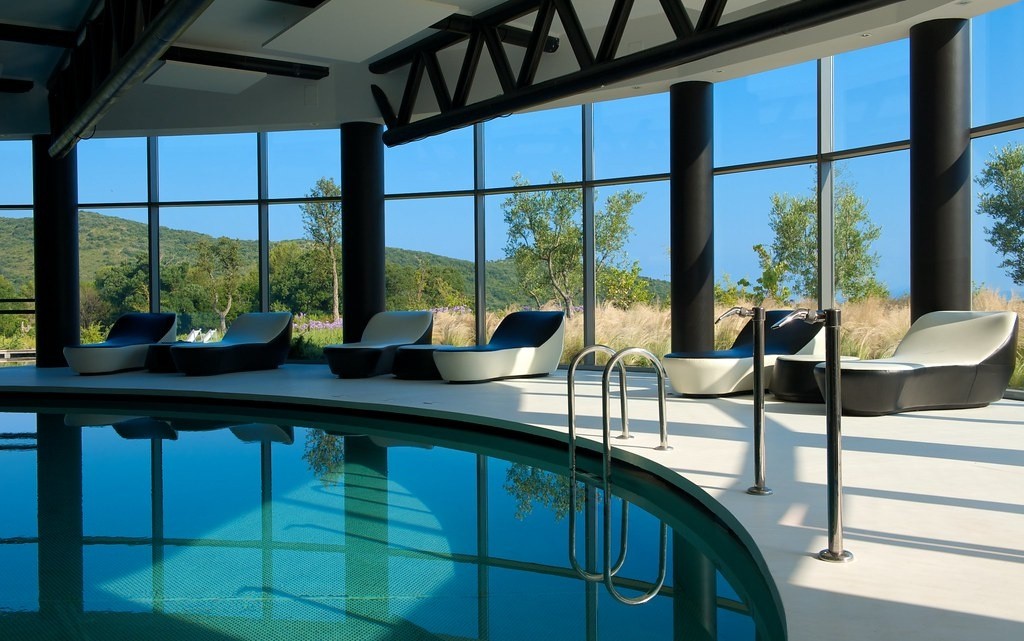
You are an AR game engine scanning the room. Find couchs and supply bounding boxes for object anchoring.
[170,311,292,373]
[813,311,1019,416]
[63,310,178,373]
[434,310,565,381]
[324,311,434,376]
[663,310,826,397]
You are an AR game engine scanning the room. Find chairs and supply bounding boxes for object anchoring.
[184,329,217,343]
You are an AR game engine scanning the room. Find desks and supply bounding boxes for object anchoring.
[391,345,454,381]
[770,356,858,403]
[145,340,189,373]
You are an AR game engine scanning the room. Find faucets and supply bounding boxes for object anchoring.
[771,309,859,565]
[715,306,772,496]
